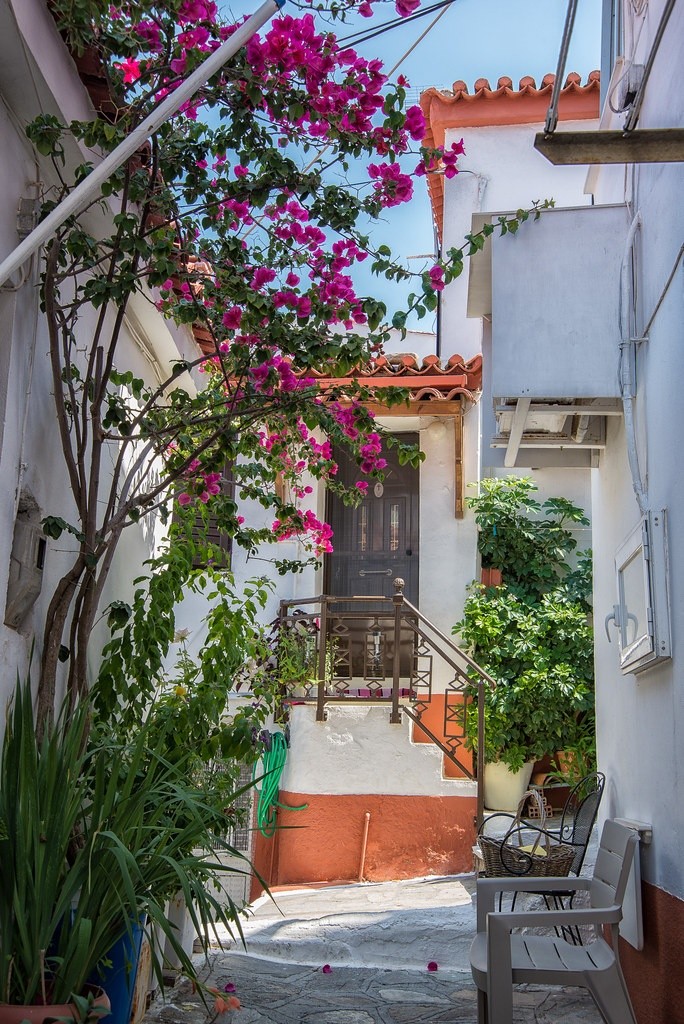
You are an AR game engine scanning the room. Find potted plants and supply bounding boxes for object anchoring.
[547,707,595,784]
[465,474,590,587]
[451,581,594,812]
[1,624,340,1023]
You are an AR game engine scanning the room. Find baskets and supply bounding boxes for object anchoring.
[478,789,576,877]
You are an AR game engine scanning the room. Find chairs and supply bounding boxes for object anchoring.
[469,819,640,1022]
[477,771,606,946]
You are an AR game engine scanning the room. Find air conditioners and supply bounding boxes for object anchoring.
[494,397,574,439]
